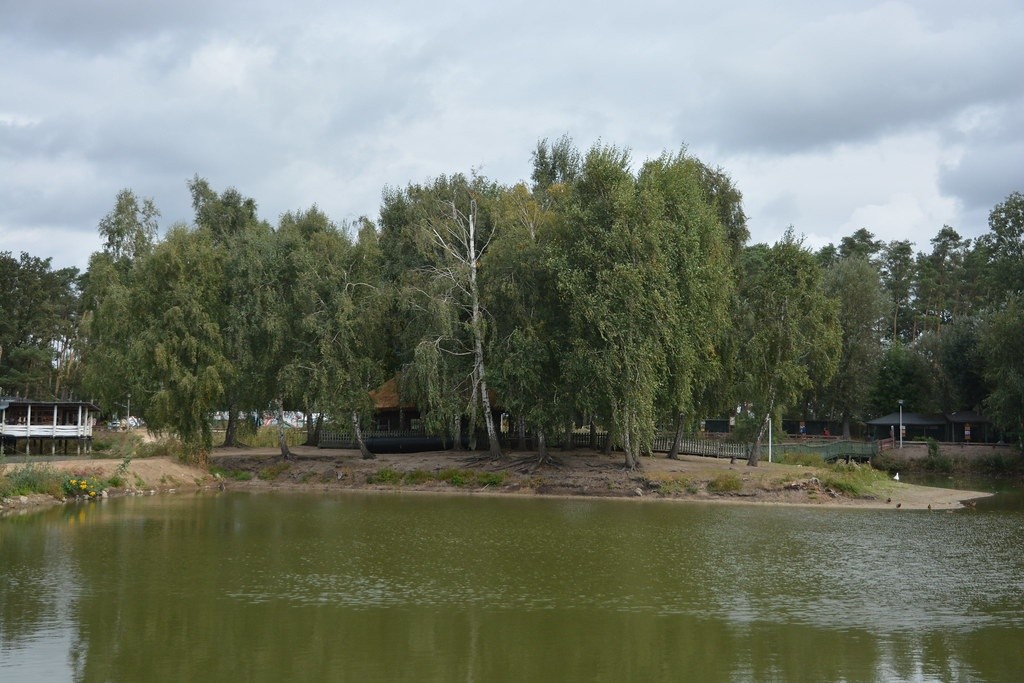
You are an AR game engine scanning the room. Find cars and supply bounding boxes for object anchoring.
[207,410,330,427]
[106,416,145,431]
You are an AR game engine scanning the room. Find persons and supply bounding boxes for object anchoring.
[802,425,807,435]
[823,428,830,439]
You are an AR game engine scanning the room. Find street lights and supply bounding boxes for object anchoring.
[896,398,905,448]
[113,394,131,431]
